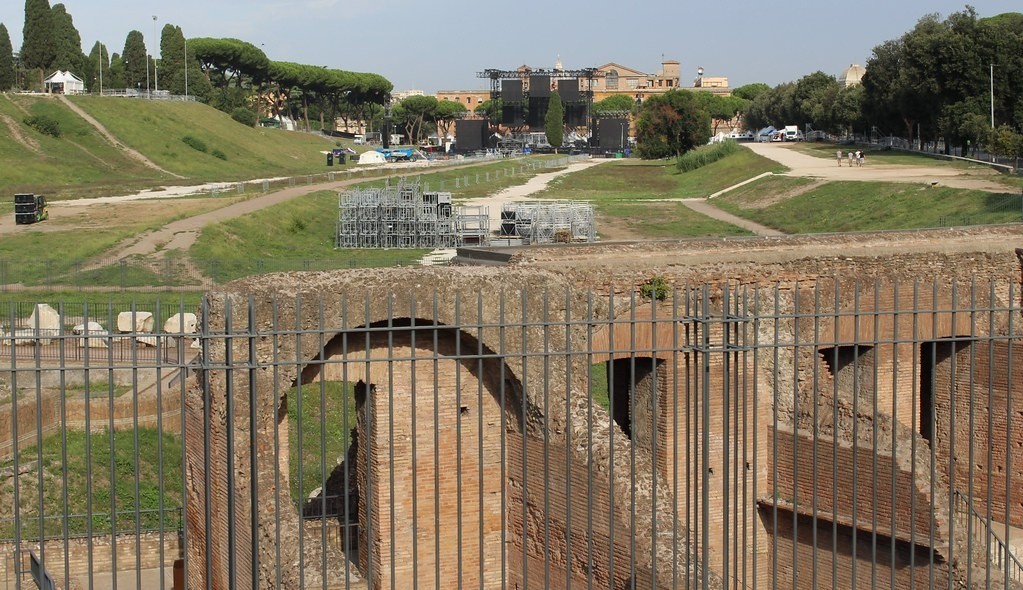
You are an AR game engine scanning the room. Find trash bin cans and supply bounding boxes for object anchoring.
[339,152,347,164]
[326,153,334,166]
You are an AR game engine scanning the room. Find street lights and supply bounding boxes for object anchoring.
[990,64,1000,129]
[99,41,103,95]
[620,123,625,148]
[153,15,158,92]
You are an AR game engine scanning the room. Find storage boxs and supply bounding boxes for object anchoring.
[15,193,35,224]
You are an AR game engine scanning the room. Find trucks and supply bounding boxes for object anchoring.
[354,135,366,145]
[785,125,798,142]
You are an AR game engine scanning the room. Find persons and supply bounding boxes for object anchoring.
[848,150,865,167]
[837,150,842,167]
